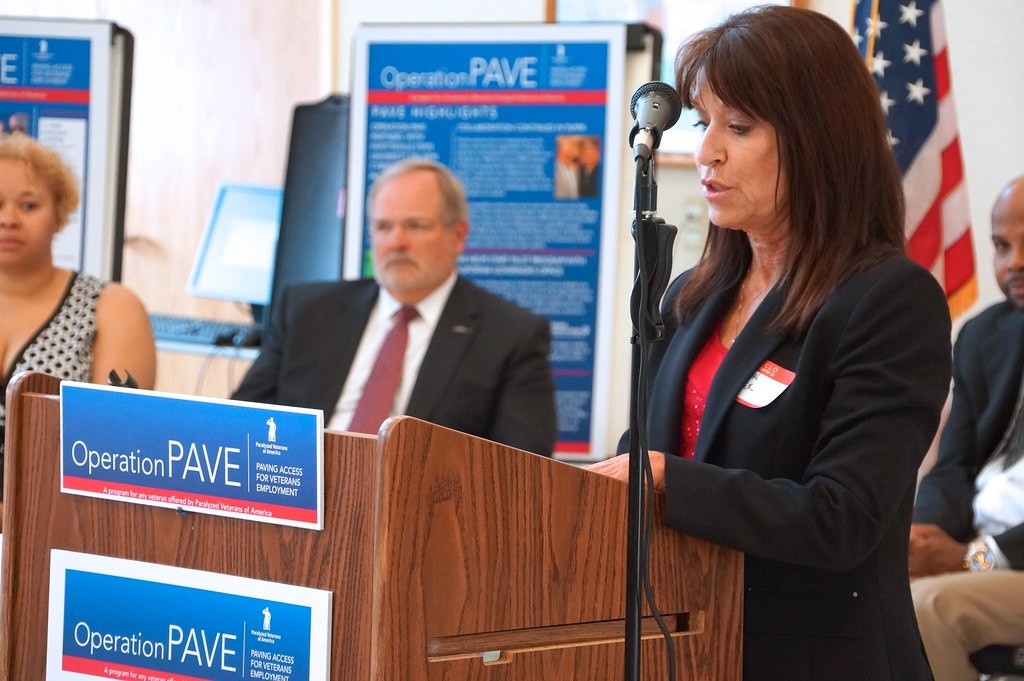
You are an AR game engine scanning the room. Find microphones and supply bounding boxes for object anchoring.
[630,81,682,164]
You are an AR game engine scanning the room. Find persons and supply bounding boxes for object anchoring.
[0,131,157,535]
[584,5,952,681]
[227,158,557,458]
[908,175,1024,681]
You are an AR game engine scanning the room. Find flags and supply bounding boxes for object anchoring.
[852,0,978,322]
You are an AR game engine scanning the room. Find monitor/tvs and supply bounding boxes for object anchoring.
[187,183,283,320]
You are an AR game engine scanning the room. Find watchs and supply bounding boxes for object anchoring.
[962,540,998,572]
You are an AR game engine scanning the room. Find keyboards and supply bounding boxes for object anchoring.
[147,311,241,345]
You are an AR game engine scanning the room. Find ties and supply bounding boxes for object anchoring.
[346,305,423,435]
[986,378,1024,472]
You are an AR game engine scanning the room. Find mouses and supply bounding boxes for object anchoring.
[234,325,263,347]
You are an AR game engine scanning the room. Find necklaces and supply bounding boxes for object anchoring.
[732,263,771,343]
